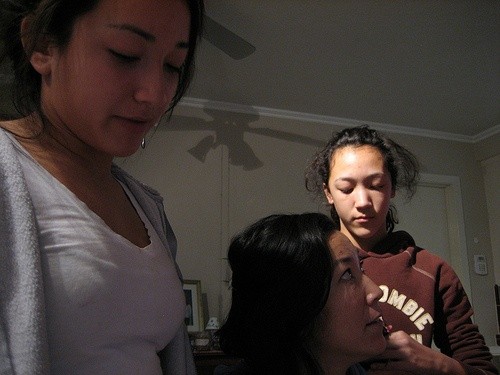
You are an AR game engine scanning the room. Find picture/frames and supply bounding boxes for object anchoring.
[182,280,204,334]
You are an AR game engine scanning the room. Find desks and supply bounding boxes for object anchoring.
[189,348,226,375]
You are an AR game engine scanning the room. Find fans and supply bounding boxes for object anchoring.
[201,14,257,62]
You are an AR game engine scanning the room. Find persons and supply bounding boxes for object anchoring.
[211,212,391,375]
[305,120,499,375]
[1,0,206,375]
